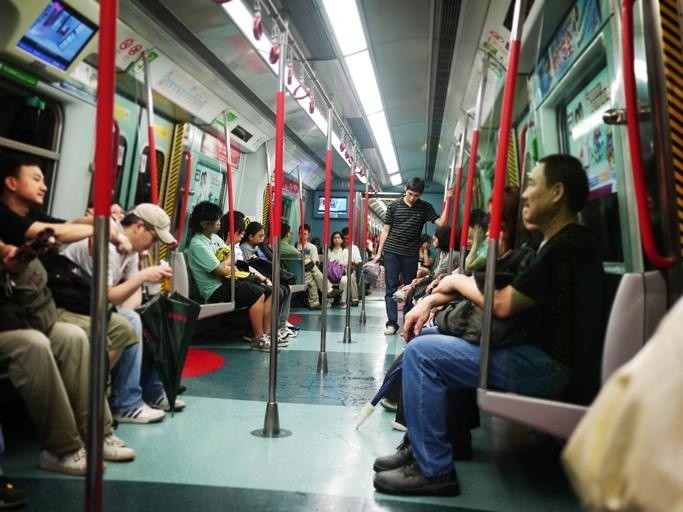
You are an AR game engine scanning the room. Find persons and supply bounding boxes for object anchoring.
[187,200,300,351]
[278,221,361,308]
[365,174,487,338]
[367,151,611,496]
[0,157,138,478]
[381,183,540,430]
[0,235,30,511]
[47,201,188,422]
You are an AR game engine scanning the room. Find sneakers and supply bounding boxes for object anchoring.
[310,289,359,311]
[38,384,186,476]
[244,320,300,352]
[384,325,395,335]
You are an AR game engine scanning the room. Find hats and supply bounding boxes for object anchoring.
[130,201,177,245]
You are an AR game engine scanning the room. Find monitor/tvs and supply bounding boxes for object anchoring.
[5,0,100,83]
[313,191,349,218]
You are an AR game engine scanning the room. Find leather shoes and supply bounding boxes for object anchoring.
[373,396,473,496]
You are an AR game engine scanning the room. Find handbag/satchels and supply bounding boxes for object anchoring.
[434,286,528,350]
[413,277,432,299]
[215,247,261,286]
[249,258,297,285]
[0,256,56,333]
[328,261,345,283]
[42,255,92,317]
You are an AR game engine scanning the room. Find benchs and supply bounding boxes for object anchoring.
[172,246,346,319]
[477,269,667,440]
